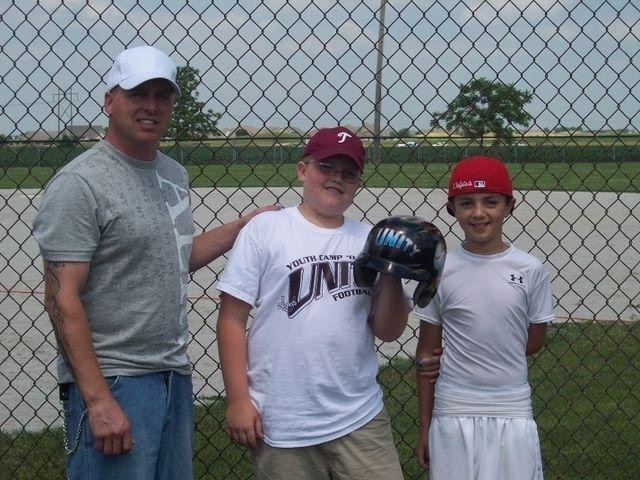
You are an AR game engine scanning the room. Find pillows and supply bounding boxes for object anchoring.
[350,209,447,309]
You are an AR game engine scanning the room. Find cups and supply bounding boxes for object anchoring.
[304,157,364,184]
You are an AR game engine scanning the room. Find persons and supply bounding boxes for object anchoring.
[414,154,554,480]
[37,43,288,478]
[215,125,415,478]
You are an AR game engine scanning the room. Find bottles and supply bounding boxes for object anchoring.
[444,153,516,219]
[301,125,367,175]
[102,44,185,102]
